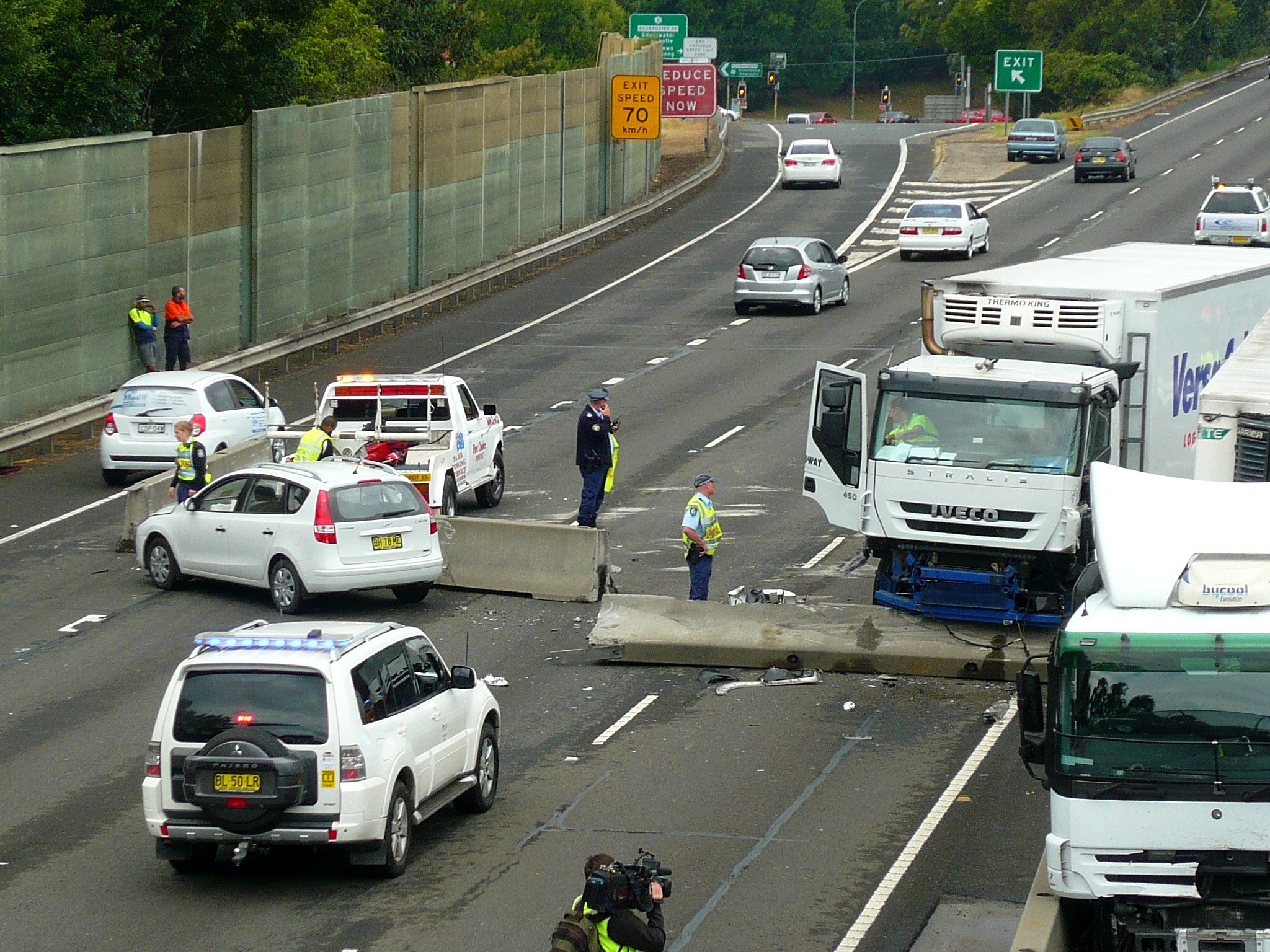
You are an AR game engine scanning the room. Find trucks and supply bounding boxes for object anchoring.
[800,241,1270,629]
[1013,302,1270,952]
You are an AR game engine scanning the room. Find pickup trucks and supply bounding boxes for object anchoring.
[309,373,505,517]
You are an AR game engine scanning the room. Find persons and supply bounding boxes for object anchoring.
[293,415,337,463]
[255,484,282,512]
[129,294,162,373]
[163,286,194,371]
[571,854,666,952]
[884,397,945,446]
[681,473,723,600]
[168,421,205,581]
[575,387,619,531]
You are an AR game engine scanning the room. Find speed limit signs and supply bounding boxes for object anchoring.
[609,74,661,141]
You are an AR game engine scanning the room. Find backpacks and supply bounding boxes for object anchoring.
[551,900,613,952]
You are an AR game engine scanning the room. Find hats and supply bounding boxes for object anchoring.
[588,387,609,401]
[694,474,716,488]
[135,294,150,302]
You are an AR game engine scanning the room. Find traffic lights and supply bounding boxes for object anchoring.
[767,71,775,85]
[737,84,746,99]
[881,90,890,104]
[955,72,962,86]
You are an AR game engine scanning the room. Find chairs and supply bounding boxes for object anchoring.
[249,484,281,513]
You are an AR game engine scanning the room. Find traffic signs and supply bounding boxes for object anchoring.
[718,62,763,77]
[994,49,1044,91]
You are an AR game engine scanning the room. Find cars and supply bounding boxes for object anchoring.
[875,110,922,124]
[787,112,837,124]
[1006,118,1066,162]
[1073,136,1138,183]
[898,198,991,261]
[944,107,1013,123]
[779,138,847,189]
[100,369,286,486]
[719,107,740,123]
[733,236,850,314]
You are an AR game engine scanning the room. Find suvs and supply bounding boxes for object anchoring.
[140,617,501,878]
[134,455,446,615]
[1194,174,1270,248]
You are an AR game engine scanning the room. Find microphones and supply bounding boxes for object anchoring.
[648,868,672,875]
